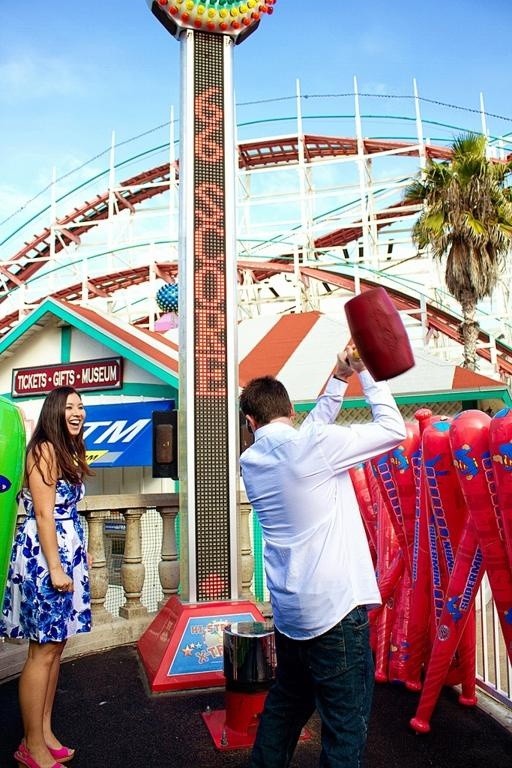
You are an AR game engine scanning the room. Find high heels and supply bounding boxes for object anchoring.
[12,736,75,767]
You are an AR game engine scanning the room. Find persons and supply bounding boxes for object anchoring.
[236,342,409,767]
[0,384,100,768]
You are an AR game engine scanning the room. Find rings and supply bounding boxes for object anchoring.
[57,587,63,593]
